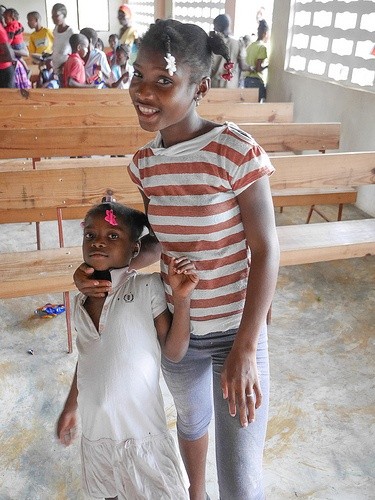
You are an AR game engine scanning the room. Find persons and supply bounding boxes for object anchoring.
[0,4,269,102]
[55,201,200,500]
[71,19,280,500]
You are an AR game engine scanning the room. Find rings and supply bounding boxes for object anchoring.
[247,394,254,397]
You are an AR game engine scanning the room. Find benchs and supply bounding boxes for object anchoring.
[0,88,375,354]
